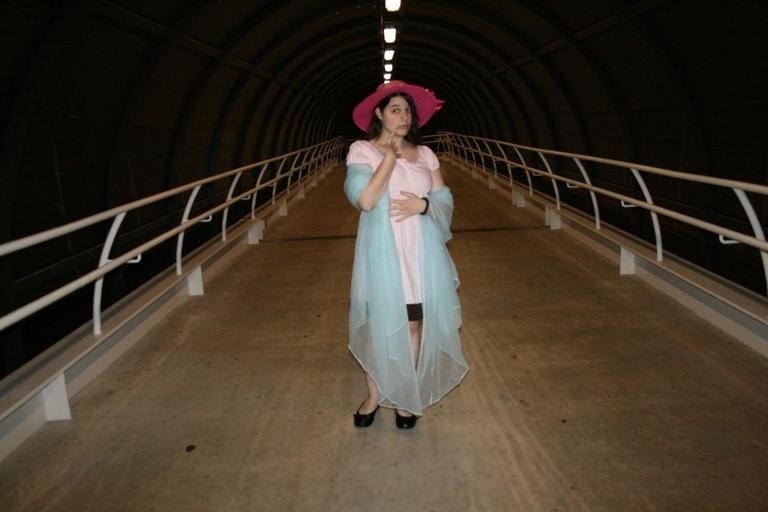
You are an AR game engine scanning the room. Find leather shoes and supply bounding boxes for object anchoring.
[353,399,380,427]
[395,409,416,429]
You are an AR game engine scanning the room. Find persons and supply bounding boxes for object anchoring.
[344,80,470,429]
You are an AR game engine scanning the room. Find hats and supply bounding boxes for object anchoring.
[351,80,445,134]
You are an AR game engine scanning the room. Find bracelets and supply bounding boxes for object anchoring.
[421,198,429,215]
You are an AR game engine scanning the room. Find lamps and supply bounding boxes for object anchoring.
[383,0,402,15]
[382,63,394,85]
[382,48,395,61]
[382,26,397,44]
